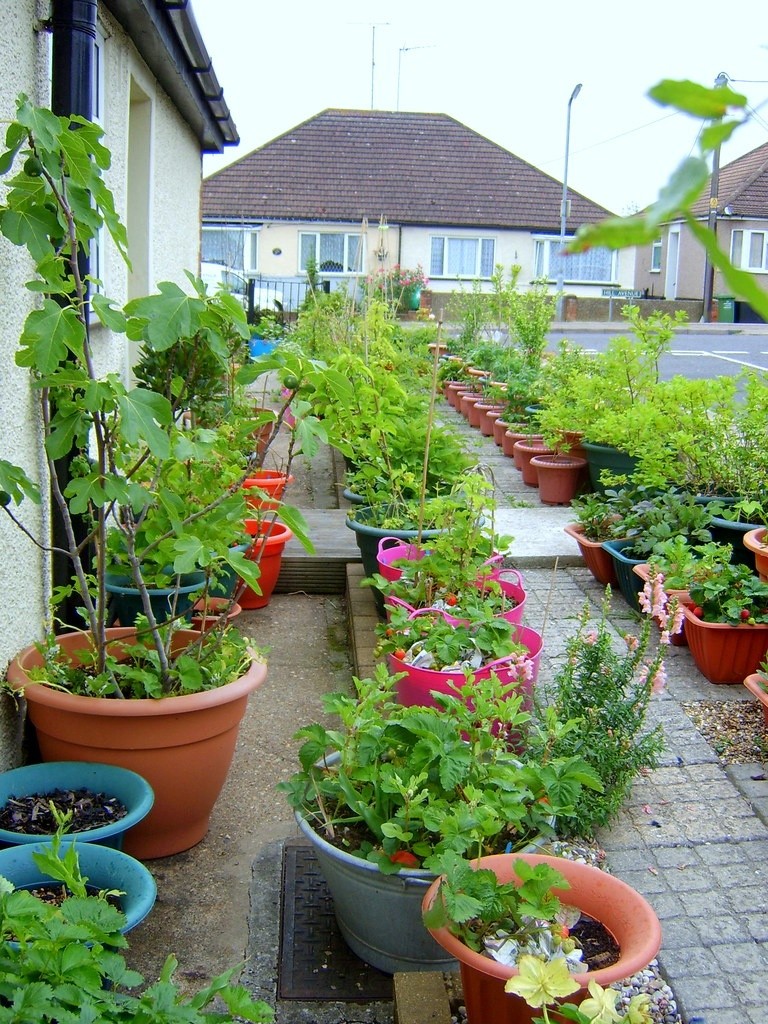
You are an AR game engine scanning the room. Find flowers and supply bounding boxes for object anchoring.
[376,263,428,292]
[504,955,654,1024]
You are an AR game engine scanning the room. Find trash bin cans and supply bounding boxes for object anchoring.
[713,295,736,323]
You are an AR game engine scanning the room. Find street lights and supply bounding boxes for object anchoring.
[553,84,585,317]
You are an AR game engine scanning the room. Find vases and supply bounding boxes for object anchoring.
[404,288,421,310]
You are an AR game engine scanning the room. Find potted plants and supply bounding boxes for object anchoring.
[0,88,768,1024]
